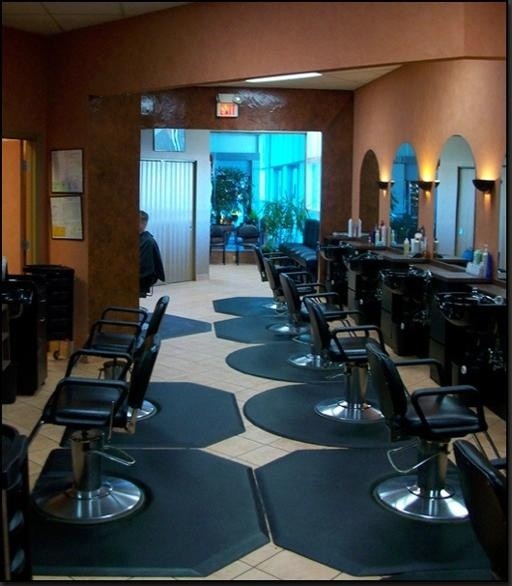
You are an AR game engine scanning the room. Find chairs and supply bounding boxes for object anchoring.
[367,341,489,524]
[235,222,263,266]
[304,296,388,424]
[254,246,312,310]
[72,295,169,424]
[263,258,331,335]
[280,273,360,369]
[210,224,228,265]
[26,337,163,525]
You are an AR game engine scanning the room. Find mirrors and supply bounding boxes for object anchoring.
[357,149,378,234]
[433,135,476,263]
[389,141,420,249]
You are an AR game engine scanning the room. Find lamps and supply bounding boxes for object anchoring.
[378,178,395,192]
[415,178,439,191]
[474,174,498,195]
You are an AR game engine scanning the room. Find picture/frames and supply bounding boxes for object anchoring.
[51,148,83,194]
[51,194,85,241]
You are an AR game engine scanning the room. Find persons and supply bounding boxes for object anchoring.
[138,210,166,298]
